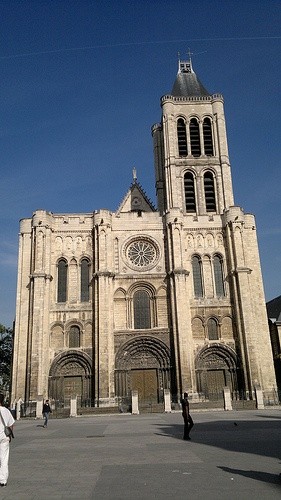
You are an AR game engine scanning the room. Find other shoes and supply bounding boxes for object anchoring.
[0,483,8,488]
[43,425,48,428]
[183,437,191,440]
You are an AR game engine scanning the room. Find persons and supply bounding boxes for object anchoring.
[43,400,53,428]
[182,392,194,440]
[0,394,16,487]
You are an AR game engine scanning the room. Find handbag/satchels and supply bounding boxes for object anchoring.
[5,427,14,440]
